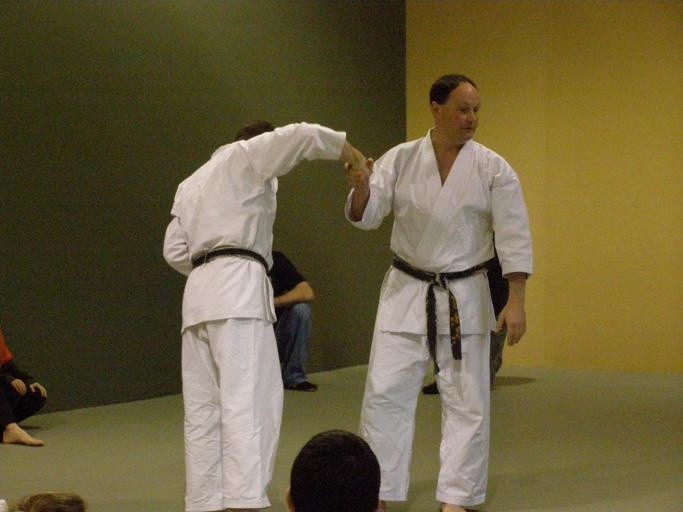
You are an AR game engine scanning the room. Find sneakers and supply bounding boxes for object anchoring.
[285,379,317,391]
[423,381,440,394]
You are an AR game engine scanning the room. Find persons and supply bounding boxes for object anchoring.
[342,73,533,512]
[163,119,372,512]
[0,329,47,447]
[9,491,89,512]
[284,428,382,512]
[266,250,319,392]
[422,256,510,395]
